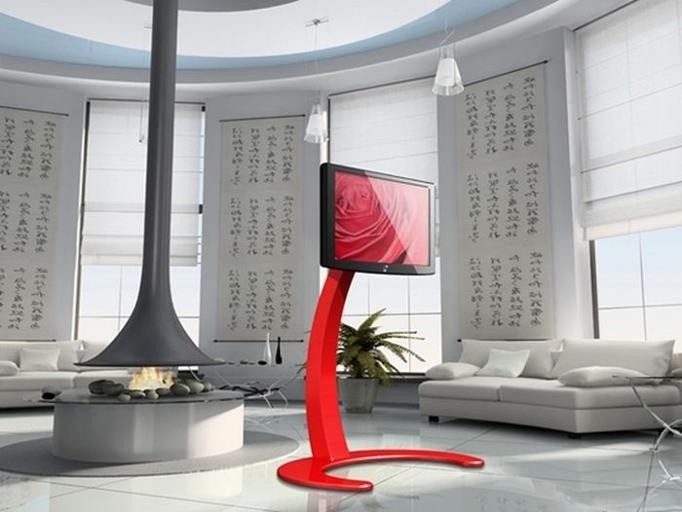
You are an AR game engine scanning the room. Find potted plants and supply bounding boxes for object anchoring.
[285,307,426,414]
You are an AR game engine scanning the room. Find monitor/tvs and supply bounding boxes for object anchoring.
[320,162,437,275]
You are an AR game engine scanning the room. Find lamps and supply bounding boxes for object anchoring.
[431,13,465,97]
[304,20,329,144]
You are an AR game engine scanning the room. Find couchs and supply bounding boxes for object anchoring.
[0,340,179,408]
[418,338,682,440]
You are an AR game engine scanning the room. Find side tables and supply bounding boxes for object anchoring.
[608,374,682,511]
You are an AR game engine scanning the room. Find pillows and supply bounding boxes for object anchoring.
[425,339,676,387]
[0,339,178,376]
